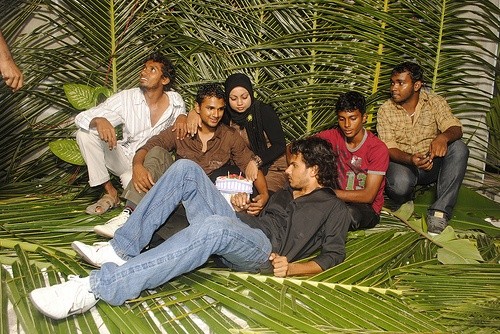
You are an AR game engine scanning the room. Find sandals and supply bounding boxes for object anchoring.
[86,190,120,215]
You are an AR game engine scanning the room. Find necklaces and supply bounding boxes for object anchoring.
[410,112,415,117]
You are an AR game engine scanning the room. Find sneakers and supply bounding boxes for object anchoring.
[94,208,132,238]
[425,209,448,236]
[71,240,127,267]
[29,274,100,320]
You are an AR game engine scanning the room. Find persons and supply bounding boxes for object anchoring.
[376,61,469,234]
[0,34,23,91]
[30,137,350,320]
[93,73,285,253]
[286,91,389,231]
[75,55,190,217]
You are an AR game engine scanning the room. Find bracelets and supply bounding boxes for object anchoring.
[250,155,262,166]
